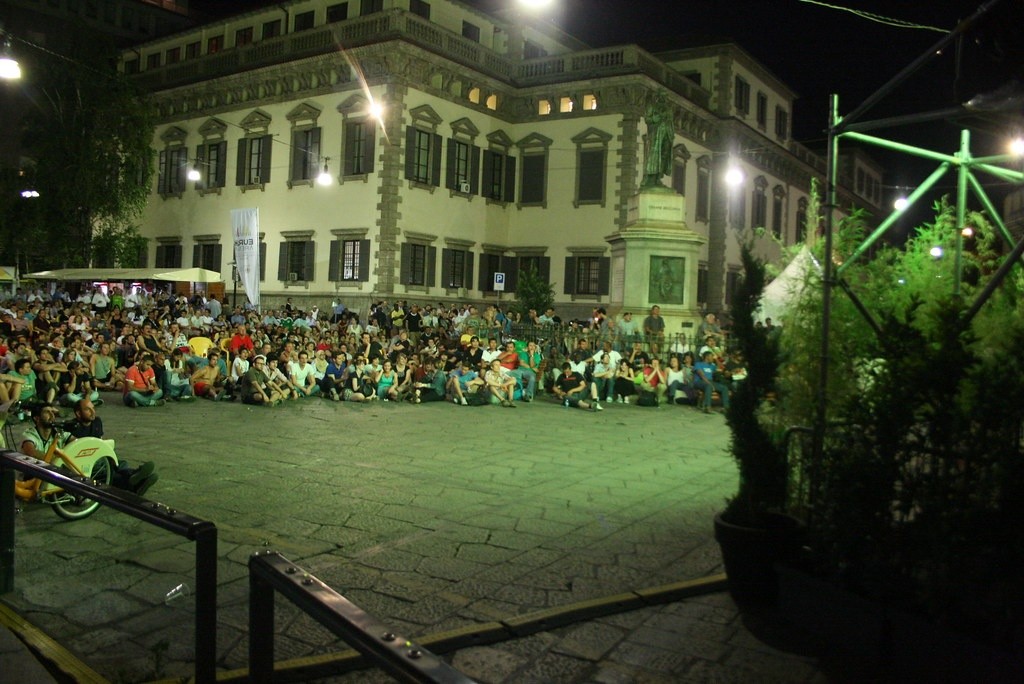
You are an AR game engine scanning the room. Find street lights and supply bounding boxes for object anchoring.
[228,260,239,309]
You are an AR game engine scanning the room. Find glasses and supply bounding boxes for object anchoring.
[256,362,265,366]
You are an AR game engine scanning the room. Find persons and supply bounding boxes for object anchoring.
[59,399,159,497]
[0,283,773,425]
[12,403,77,482]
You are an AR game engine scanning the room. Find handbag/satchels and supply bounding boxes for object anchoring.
[361,379,373,397]
[638,390,658,407]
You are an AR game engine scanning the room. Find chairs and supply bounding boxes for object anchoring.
[221,337,233,367]
[187,337,214,359]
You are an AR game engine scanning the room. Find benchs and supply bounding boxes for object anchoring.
[696,389,775,411]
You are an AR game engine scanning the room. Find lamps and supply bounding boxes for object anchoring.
[0,38,20,70]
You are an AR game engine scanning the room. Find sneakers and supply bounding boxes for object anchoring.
[92,399,104,406]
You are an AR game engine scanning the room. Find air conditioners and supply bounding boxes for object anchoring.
[290,273,298,281]
[253,176,260,184]
[460,183,470,193]
[457,288,468,298]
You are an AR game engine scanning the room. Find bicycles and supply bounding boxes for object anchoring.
[1,403,120,521]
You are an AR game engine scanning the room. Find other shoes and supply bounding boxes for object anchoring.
[523,395,532,402]
[214,389,226,401]
[154,400,166,406]
[129,461,159,496]
[501,400,516,407]
[223,395,237,402]
[362,396,377,404]
[333,394,339,401]
[577,400,589,409]
[704,405,712,413]
[412,393,417,402]
[591,402,603,410]
[454,394,458,403]
[263,400,273,408]
[690,399,697,406]
[667,395,675,404]
[180,395,196,403]
[597,397,600,401]
[617,396,629,404]
[274,399,282,405]
[460,397,468,406]
[606,396,613,403]
